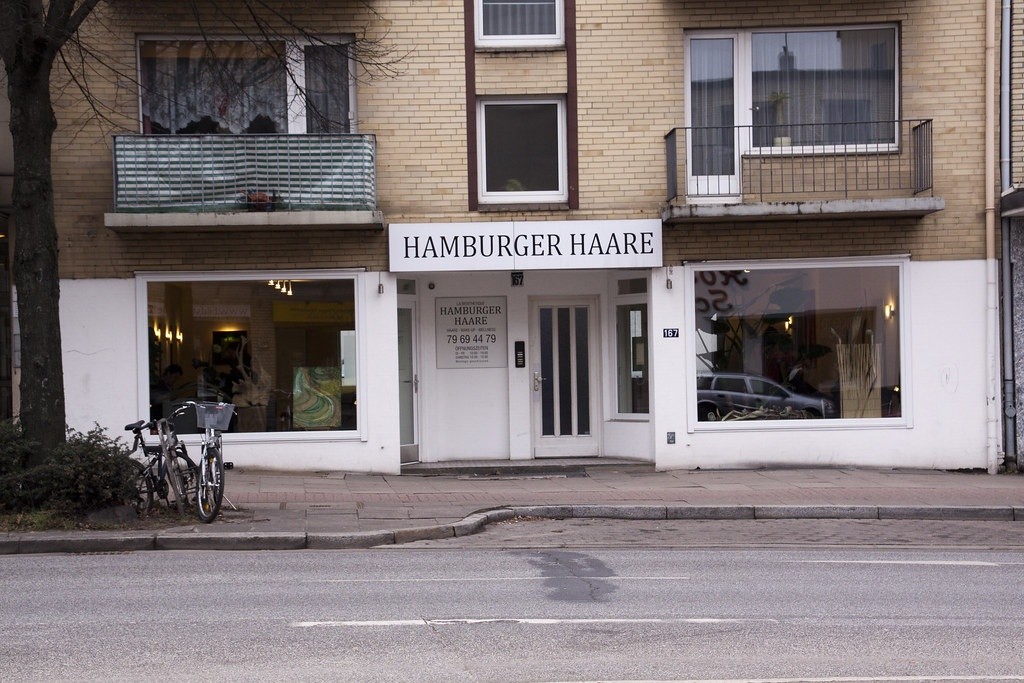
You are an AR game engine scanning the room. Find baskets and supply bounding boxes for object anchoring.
[195,404,234,430]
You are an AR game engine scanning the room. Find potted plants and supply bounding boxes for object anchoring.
[767,89,794,149]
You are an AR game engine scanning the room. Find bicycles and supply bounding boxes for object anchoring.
[124,405,200,516]
[173,400,238,523]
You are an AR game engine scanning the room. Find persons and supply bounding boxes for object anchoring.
[157,365,199,433]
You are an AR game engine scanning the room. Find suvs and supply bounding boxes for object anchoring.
[697,372,835,421]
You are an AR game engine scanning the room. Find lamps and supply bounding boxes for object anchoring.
[268,280,293,296]
[885,305,895,321]
